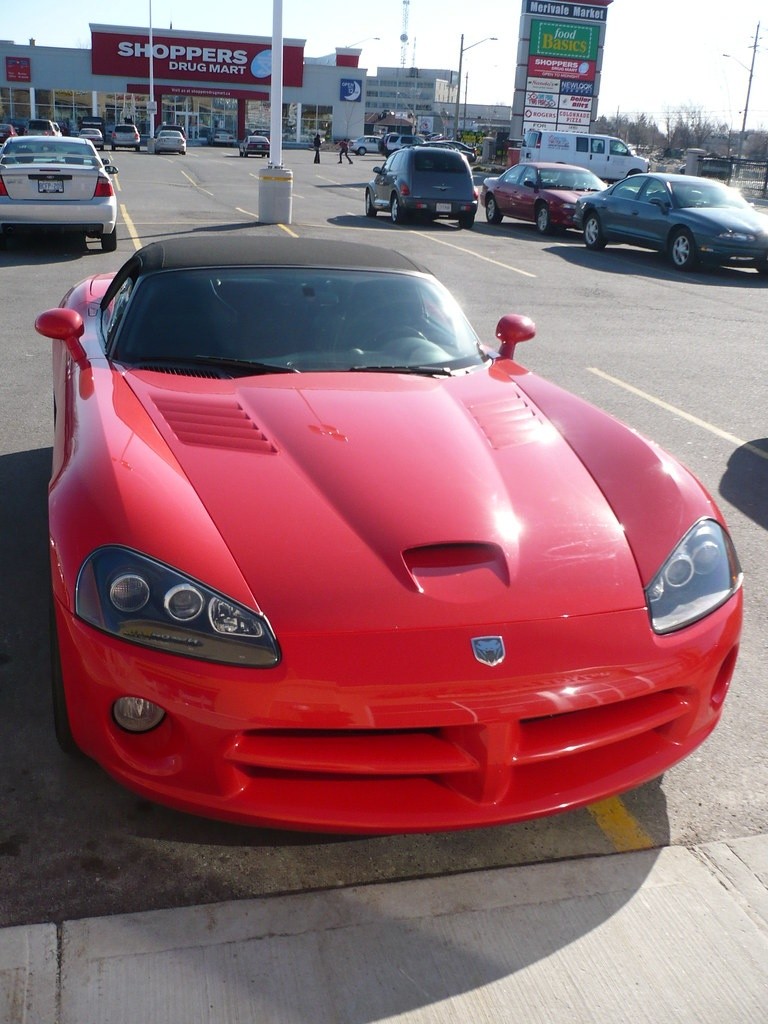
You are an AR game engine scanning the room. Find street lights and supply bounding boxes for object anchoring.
[722,53,753,180]
[452,34,499,142]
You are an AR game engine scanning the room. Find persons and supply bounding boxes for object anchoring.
[335,141,353,164]
[314,134,321,163]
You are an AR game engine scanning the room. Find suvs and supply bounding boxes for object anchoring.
[108,123,142,152]
[160,125,187,141]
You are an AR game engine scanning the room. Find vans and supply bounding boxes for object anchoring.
[519,129,652,184]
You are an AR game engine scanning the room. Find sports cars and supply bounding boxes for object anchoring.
[34,231,745,836]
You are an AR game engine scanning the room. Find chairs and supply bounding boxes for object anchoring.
[211,278,279,360]
[66,151,84,164]
[597,143,602,152]
[15,148,34,163]
[334,280,412,353]
[689,191,702,202]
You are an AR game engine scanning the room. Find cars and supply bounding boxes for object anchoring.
[479,161,611,236]
[0,123,19,144]
[679,158,732,180]
[569,171,768,279]
[364,146,479,229]
[238,135,270,159]
[250,129,270,143]
[23,115,106,137]
[349,135,382,156]
[153,130,187,155]
[0,135,118,252]
[189,123,328,142]
[75,128,105,151]
[6,118,28,135]
[208,130,236,148]
[382,130,480,164]
